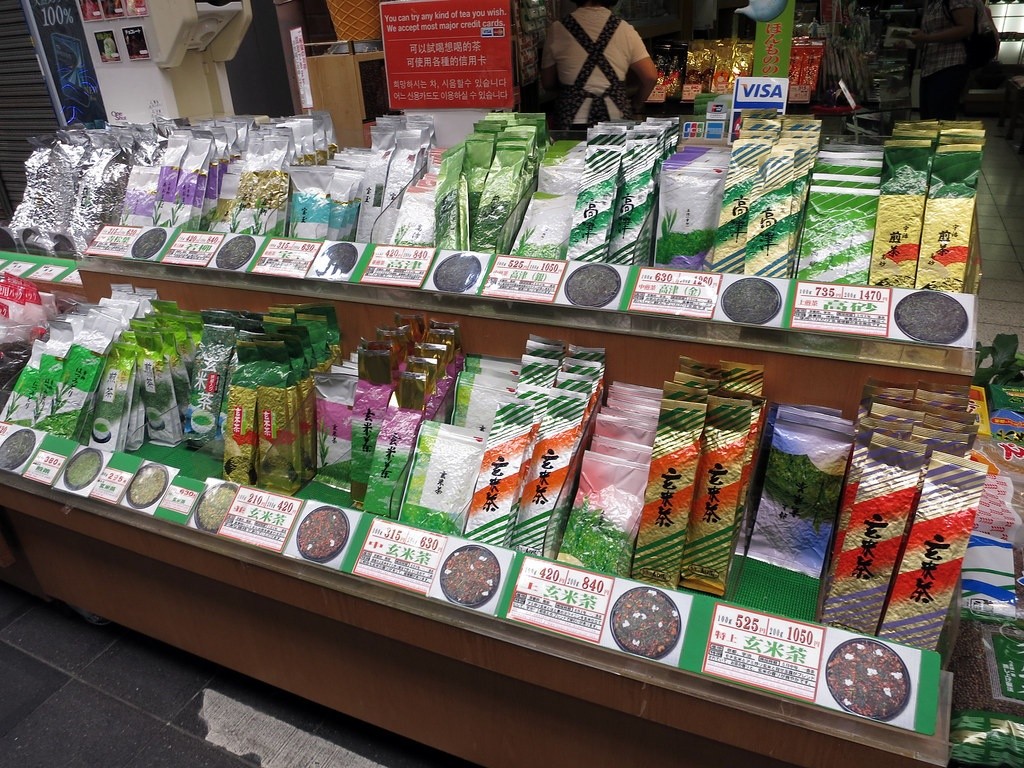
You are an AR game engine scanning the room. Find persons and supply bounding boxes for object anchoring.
[906,0,983,127]
[540,0,659,138]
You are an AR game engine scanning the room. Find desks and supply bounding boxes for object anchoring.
[302,49,393,148]
